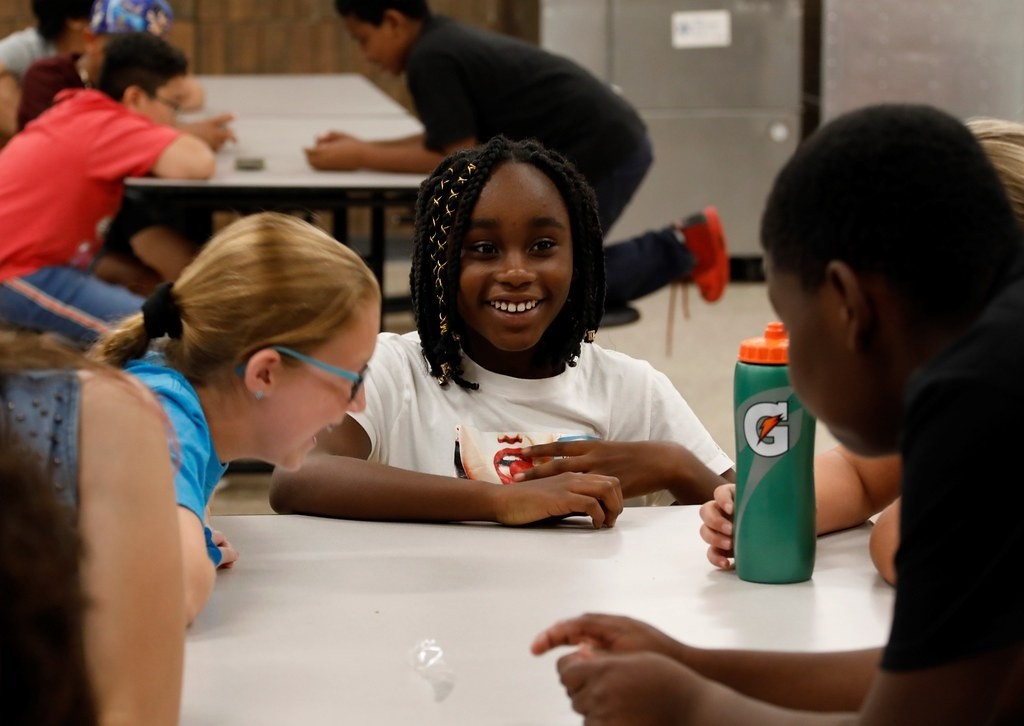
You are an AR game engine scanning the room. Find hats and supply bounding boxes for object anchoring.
[90,0,174,38]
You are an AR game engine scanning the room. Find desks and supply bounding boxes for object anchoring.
[123,73,428,334]
[181,503,898,726]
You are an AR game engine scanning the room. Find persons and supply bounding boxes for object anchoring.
[699,116,1024,589]
[530,99,1024,726]
[0,321,189,726]
[17,0,174,130]
[0,28,240,352]
[0,0,87,73]
[302,0,731,309]
[27,208,384,632]
[267,133,737,528]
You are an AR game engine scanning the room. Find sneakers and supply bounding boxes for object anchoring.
[680,206,729,302]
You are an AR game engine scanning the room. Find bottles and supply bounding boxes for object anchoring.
[734,321,818,583]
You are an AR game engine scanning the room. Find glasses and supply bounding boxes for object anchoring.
[145,92,183,114]
[234,345,369,402]
